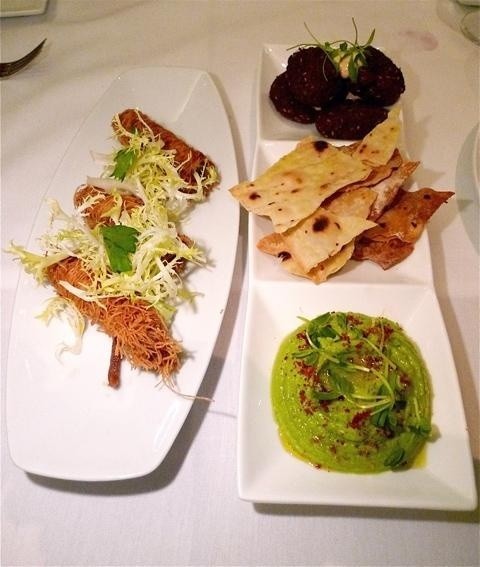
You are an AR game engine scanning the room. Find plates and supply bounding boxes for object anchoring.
[4,67,241,483]
[246,43,478,515]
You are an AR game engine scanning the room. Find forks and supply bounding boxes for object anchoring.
[1,39,45,79]
